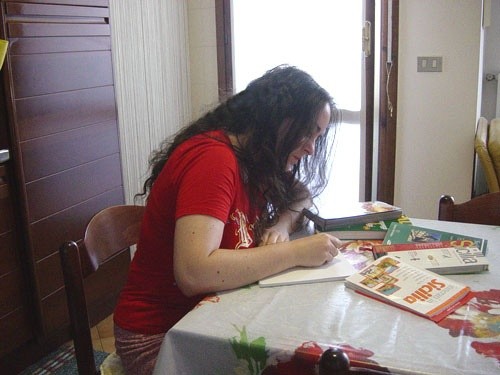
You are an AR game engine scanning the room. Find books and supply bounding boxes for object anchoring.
[259,201,490,324]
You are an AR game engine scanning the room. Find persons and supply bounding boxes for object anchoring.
[113,66,343,375]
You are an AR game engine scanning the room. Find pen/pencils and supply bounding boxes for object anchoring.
[316,229,343,257]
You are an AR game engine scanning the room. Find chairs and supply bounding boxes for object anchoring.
[58,205,145,375]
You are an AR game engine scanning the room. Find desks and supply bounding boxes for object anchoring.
[171,216,500,375]
